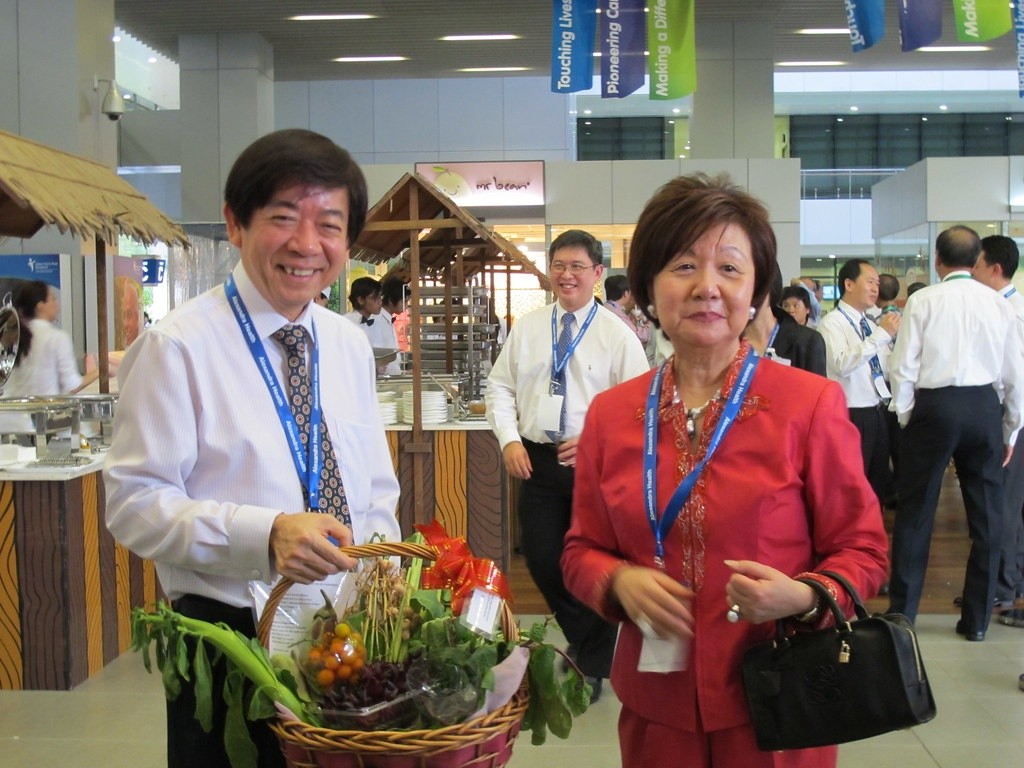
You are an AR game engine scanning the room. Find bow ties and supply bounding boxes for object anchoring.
[392,317,396,322]
[360,315,374,327]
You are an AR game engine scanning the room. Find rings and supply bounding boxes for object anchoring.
[727,604,739,623]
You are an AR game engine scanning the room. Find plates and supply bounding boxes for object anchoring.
[376,391,454,426]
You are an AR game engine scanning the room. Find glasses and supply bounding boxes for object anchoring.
[549,263,597,275]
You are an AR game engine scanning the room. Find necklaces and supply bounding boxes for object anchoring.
[671,383,723,438]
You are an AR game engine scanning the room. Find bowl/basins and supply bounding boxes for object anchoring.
[469,401,485,414]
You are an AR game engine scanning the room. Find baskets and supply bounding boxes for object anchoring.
[256,540,530,768]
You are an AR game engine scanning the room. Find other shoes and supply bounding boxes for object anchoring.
[1001,609,1024,627]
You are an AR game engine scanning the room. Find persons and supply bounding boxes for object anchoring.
[621,293,644,328]
[0,279,117,400]
[475,296,508,343]
[602,274,651,350]
[342,277,397,379]
[367,279,411,375]
[777,285,817,331]
[314,286,331,308]
[907,281,927,300]
[143,311,152,329]
[873,273,903,343]
[953,236,1024,608]
[484,230,651,706]
[425,298,459,340]
[871,225,1024,641]
[791,277,823,324]
[816,259,901,597]
[560,167,889,768]
[102,128,402,768]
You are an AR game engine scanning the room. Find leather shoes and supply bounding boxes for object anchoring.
[954,596,1013,608]
[955,619,984,641]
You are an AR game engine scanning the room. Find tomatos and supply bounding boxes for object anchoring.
[305,623,370,688]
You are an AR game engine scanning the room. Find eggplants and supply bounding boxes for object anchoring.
[314,590,338,638]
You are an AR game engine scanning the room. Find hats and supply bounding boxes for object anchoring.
[322,286,332,299]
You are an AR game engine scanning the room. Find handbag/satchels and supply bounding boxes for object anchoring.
[741,569,937,752]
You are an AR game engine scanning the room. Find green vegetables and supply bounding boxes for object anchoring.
[128,600,320,768]
[409,616,593,745]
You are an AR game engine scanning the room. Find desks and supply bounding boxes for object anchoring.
[385,417,516,577]
[0,441,169,691]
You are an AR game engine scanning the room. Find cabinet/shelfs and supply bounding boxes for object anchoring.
[402,284,491,407]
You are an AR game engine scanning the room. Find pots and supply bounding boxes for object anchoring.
[78,394,119,447]
[0,397,80,455]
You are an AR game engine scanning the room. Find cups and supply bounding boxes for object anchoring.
[631,309,642,325]
[554,429,577,465]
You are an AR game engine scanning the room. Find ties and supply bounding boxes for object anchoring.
[861,318,885,398]
[272,323,359,573]
[545,313,576,446]
